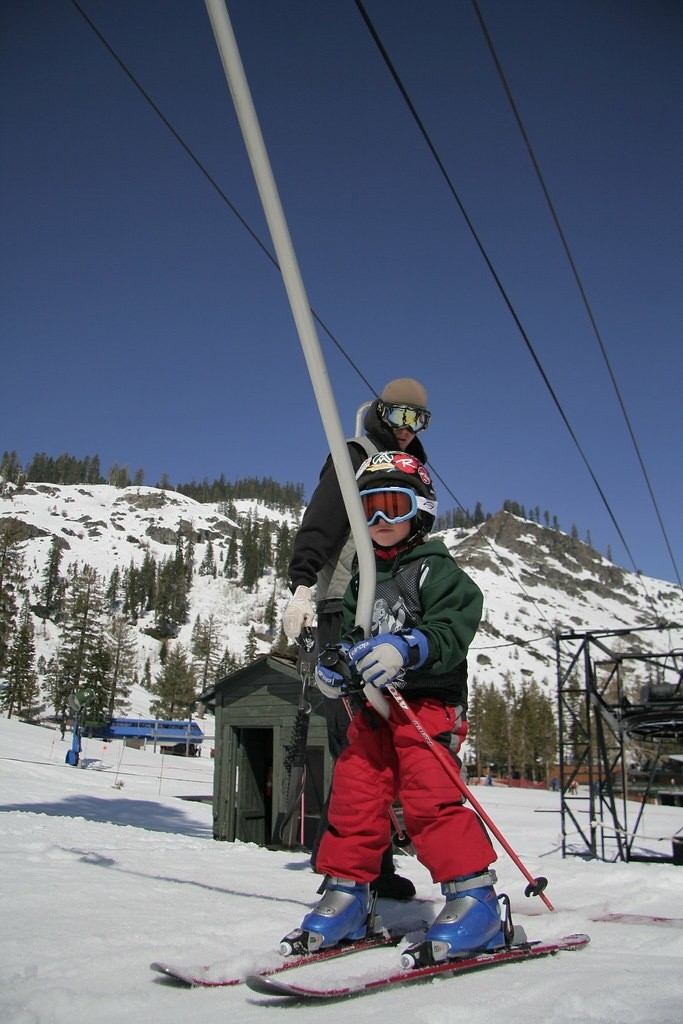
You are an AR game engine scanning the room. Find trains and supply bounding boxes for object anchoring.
[77,717,204,744]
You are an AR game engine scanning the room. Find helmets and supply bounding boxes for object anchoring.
[355,452,438,533]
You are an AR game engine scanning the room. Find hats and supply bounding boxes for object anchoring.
[383,378,429,410]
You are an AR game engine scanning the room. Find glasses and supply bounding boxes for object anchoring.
[359,486,438,527]
[379,404,431,433]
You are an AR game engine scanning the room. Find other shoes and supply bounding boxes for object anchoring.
[371,873,416,899]
[424,868,507,957]
[299,875,366,947]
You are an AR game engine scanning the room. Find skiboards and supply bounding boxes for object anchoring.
[148,918,592,999]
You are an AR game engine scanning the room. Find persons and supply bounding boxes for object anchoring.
[551,778,557,791]
[60,719,67,740]
[282,378,433,901]
[414,411,423,426]
[277,450,513,968]
[465,774,493,785]
[568,780,577,792]
[402,409,407,425]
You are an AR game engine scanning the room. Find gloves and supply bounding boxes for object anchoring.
[283,585,314,640]
[345,628,429,688]
[315,641,353,699]
